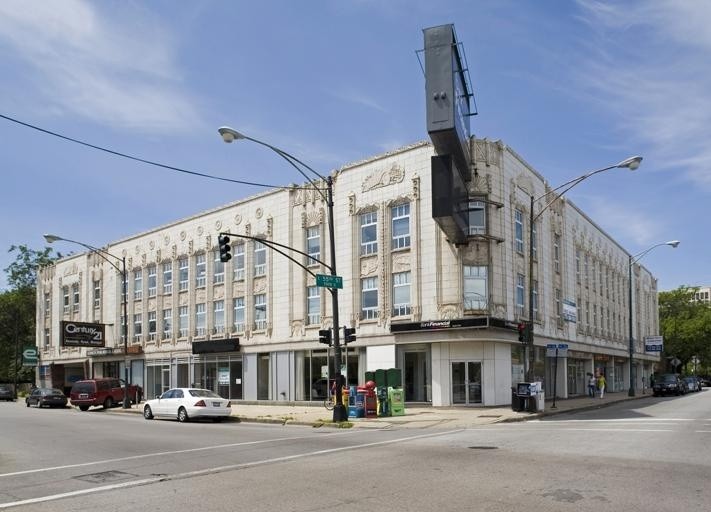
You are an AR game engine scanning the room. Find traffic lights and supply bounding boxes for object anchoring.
[219,236,232,262]
[519,322,529,343]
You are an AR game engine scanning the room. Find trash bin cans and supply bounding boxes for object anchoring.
[534,391,544,413]
[511,393,522,411]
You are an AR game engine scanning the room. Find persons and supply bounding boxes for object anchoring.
[597,372,609,399]
[650,373,656,387]
[330,379,337,394]
[588,372,597,399]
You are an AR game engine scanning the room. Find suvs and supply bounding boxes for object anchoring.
[70,377,142,411]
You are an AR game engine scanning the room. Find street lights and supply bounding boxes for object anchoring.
[217,126,347,422]
[529,156,643,382]
[629,241,680,395]
[42,233,131,409]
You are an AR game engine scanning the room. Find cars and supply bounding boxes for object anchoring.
[653,374,701,396]
[0,387,13,401]
[26,388,67,408]
[143,388,232,422]
